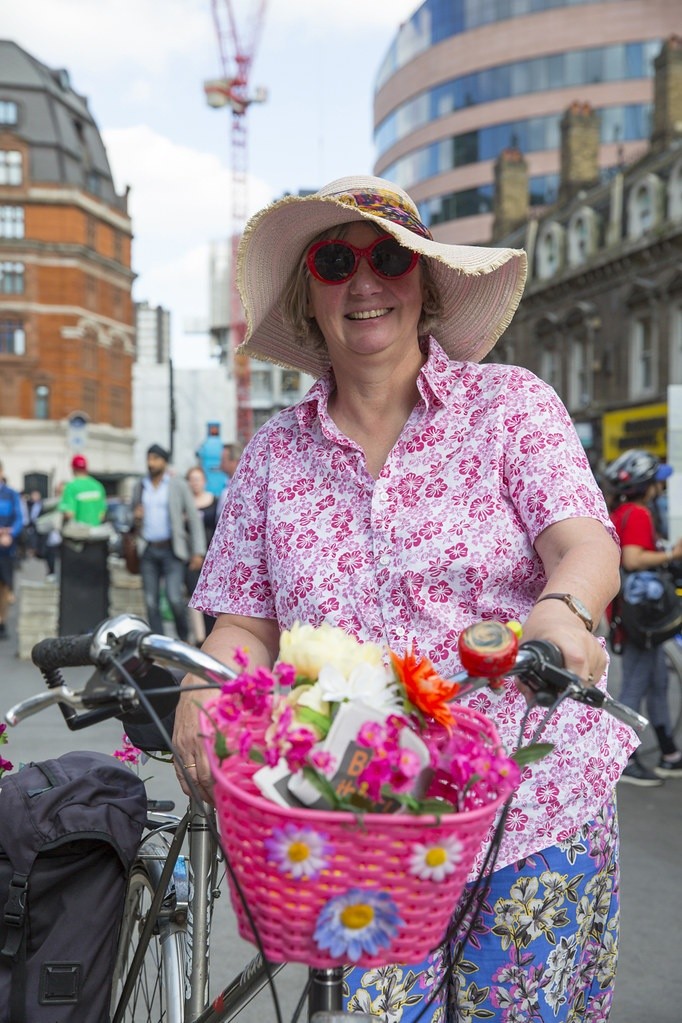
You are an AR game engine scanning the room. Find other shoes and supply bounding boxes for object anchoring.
[620,760,663,785]
[654,752,682,778]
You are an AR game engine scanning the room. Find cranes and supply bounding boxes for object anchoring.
[199,0,271,447]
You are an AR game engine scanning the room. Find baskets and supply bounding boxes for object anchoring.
[200,701,515,969]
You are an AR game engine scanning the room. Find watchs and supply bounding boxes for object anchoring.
[536,592,593,633]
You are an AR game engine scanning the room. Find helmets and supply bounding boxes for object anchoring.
[602,449,659,490]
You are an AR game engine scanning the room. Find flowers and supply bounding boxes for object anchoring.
[209,620,554,816]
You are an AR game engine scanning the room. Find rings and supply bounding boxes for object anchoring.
[183,763,196,769]
[588,673,593,682]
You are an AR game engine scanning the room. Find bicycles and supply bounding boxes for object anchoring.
[1,614,651,1023]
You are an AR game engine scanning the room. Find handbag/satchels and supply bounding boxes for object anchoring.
[610,562,681,647]
[123,526,141,573]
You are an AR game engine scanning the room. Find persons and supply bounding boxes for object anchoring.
[58,455,108,526]
[602,445,682,786]
[13,481,137,583]
[187,421,244,524]
[0,461,24,637]
[183,468,220,648]
[132,445,206,644]
[173,174,643,1023]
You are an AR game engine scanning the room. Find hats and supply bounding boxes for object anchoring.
[652,461,673,481]
[72,456,87,470]
[234,174,527,378]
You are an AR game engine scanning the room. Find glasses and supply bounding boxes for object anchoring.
[306,235,420,285]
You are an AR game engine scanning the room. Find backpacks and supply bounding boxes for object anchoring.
[0,751,144,1023]
